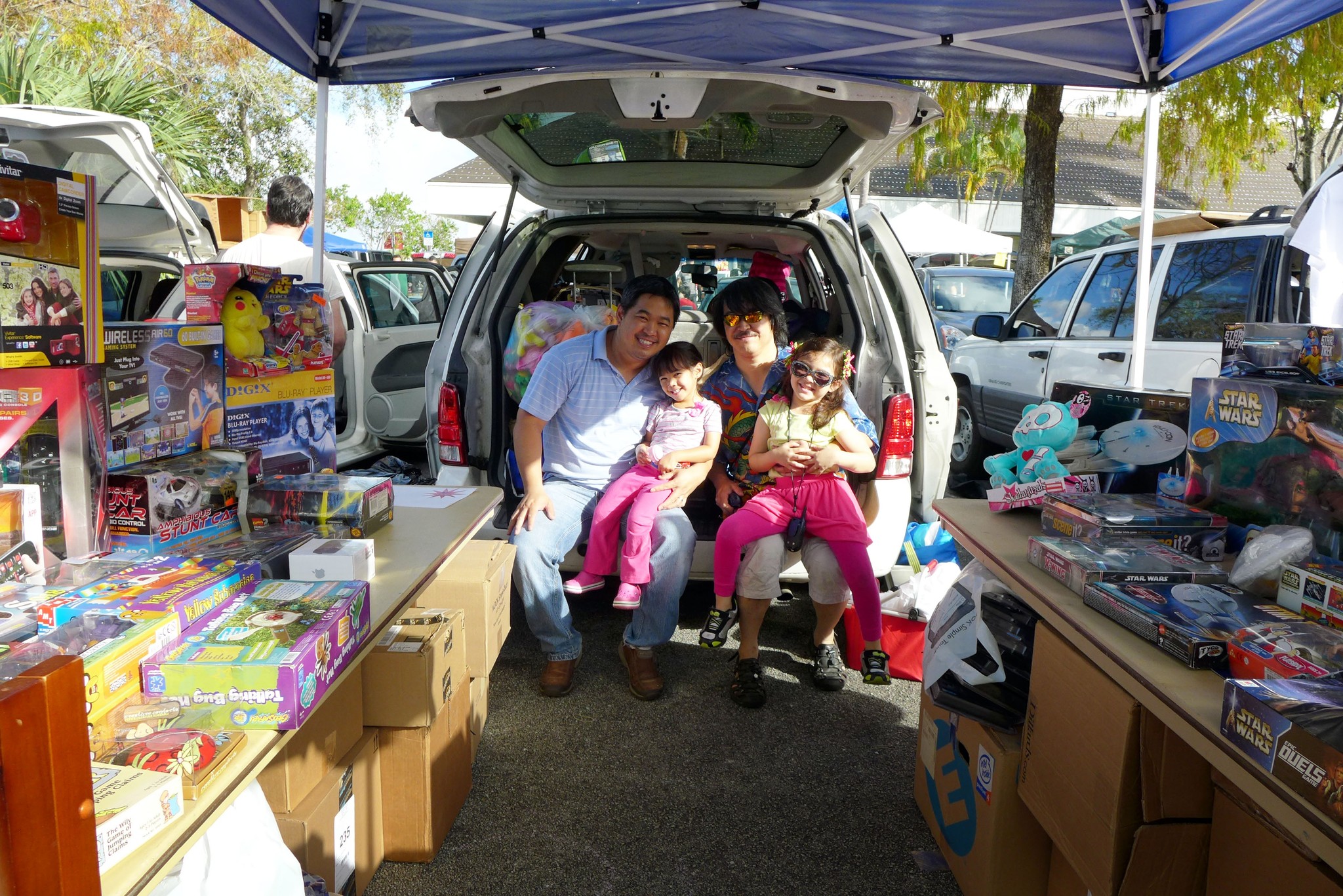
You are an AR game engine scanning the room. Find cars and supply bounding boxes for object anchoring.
[2,99,457,467]
[916,267,1018,354]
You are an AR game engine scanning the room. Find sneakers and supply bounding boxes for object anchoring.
[617,637,664,700]
[698,597,738,649]
[562,569,606,596]
[612,581,642,610]
[859,649,893,687]
[810,630,847,690]
[539,643,584,696]
[731,652,768,708]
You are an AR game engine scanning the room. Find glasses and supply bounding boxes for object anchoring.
[789,356,839,387]
[720,311,768,326]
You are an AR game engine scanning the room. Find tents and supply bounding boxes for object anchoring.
[302,224,368,258]
[196,0,1343,400]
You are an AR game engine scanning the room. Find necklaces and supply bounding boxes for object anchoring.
[786,403,822,511]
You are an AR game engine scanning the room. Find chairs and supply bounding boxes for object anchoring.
[566,262,623,310]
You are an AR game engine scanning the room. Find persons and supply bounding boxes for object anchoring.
[696,337,896,686]
[16,267,83,326]
[279,404,314,460]
[308,398,336,476]
[1303,329,1323,358]
[185,364,223,451]
[697,277,882,706]
[22,553,41,572]
[1300,345,1325,382]
[560,339,722,610]
[501,273,703,703]
[214,173,347,362]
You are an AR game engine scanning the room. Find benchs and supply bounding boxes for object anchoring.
[668,310,733,374]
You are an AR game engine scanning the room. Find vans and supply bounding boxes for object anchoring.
[402,65,960,586]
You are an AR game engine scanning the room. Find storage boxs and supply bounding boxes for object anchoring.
[0,151,524,896]
[908,319,1343,896]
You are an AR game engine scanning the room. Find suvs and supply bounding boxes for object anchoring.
[949,150,1342,495]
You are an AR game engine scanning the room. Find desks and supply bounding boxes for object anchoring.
[77,460,505,896]
[929,493,1342,883]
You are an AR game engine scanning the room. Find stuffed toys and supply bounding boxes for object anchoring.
[220,285,271,359]
[980,388,1092,491]
